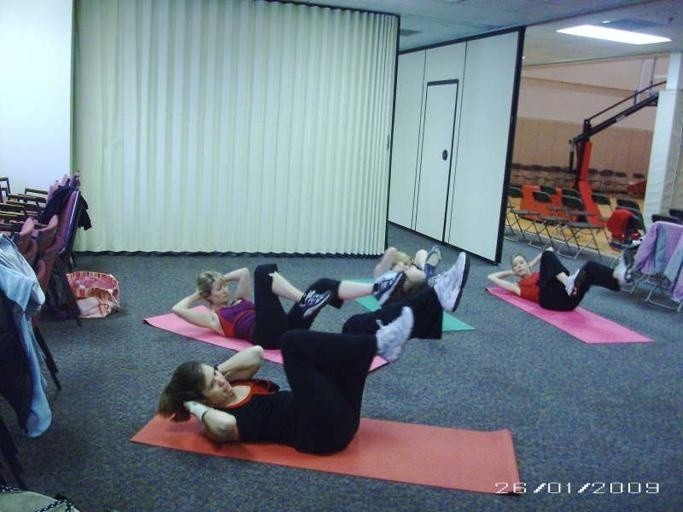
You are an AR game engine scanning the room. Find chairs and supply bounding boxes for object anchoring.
[0,164,81,490]
[501,161,683,313]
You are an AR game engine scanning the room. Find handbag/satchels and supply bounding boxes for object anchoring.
[65,271,120,318]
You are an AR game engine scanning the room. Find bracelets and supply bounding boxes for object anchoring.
[190,403,208,423]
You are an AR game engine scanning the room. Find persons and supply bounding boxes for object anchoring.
[171,263,406,350]
[373,244,443,296]
[157,249,472,456]
[487,246,634,311]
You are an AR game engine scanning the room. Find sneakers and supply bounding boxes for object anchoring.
[423,244,442,281]
[429,252,469,314]
[613,247,634,286]
[565,267,586,299]
[299,288,334,320]
[372,271,406,308]
[377,305,414,363]
